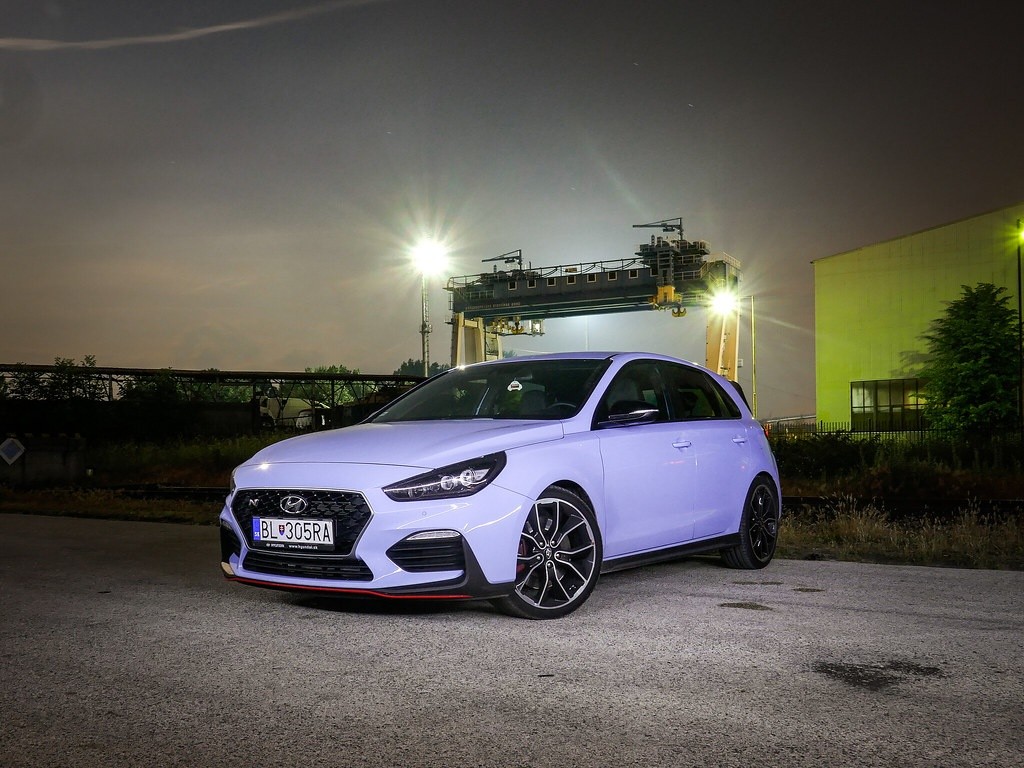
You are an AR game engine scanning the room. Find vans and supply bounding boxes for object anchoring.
[261,397,330,427]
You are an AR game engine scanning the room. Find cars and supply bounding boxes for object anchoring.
[219,348,784,619]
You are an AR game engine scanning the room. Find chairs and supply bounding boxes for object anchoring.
[459,383,497,415]
[520,390,550,415]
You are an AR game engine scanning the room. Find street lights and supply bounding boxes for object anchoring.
[712,288,743,377]
[411,236,448,378]
